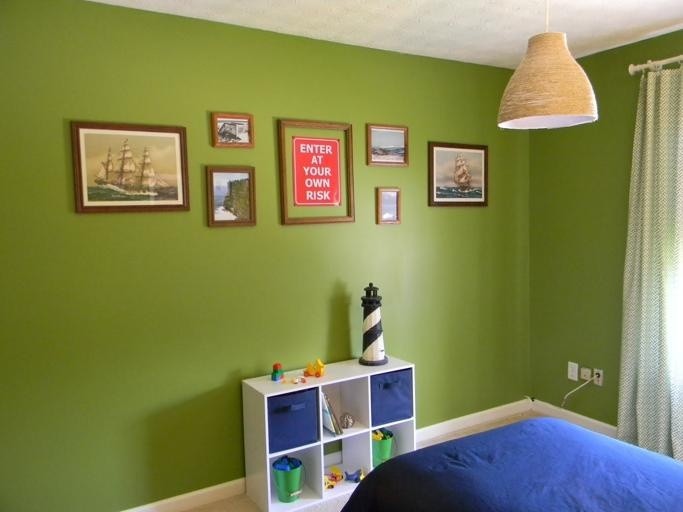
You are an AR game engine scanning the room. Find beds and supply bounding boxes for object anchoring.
[340,409,683,512]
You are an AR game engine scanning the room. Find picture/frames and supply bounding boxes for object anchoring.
[424,138,488,212]
[273,114,356,227]
[374,184,402,227]
[363,120,410,171]
[206,106,254,152]
[67,115,192,216]
[203,162,257,230]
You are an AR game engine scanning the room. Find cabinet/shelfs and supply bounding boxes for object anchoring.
[235,357,420,512]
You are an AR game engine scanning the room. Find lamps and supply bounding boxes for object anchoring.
[485,1,603,134]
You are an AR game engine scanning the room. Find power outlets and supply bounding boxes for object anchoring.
[592,369,604,387]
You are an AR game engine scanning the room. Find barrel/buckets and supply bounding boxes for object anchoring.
[372,429,399,468]
[271,456,307,504]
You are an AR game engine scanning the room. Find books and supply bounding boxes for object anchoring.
[320,390,342,439]
